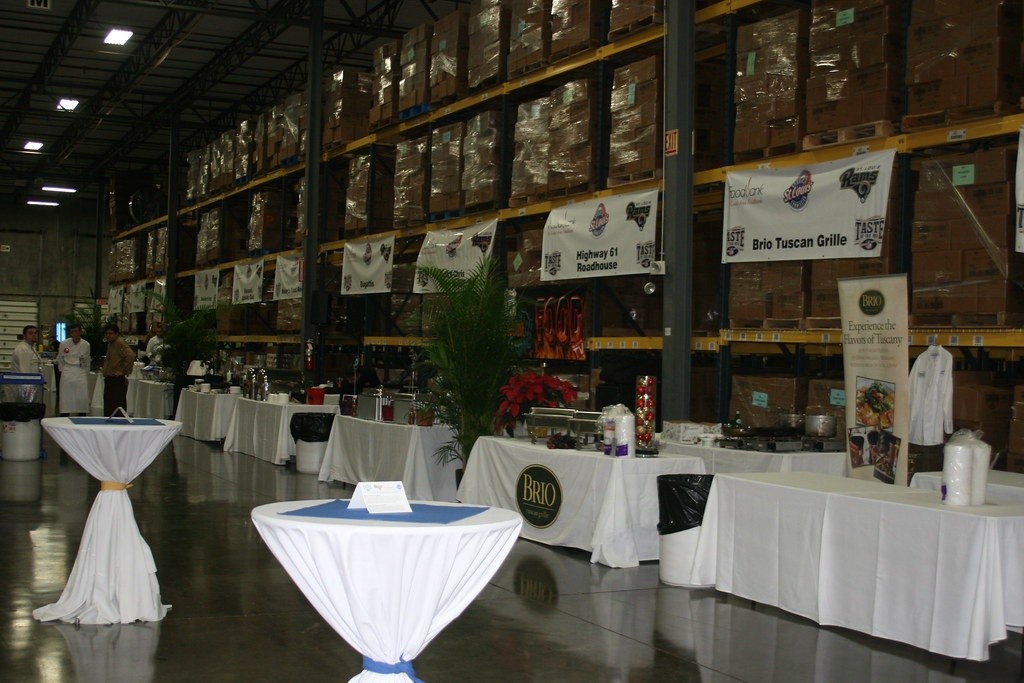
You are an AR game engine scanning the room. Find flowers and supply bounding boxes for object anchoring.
[495,374,578,437]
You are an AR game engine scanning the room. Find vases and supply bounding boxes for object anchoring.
[414,409,434,426]
[529,425,548,438]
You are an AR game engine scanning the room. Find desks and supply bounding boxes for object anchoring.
[685,473,1024,661]
[33,416,184,625]
[317,414,460,502]
[455,436,705,568]
[251,499,523,682]
[909,470,1024,503]
[222,397,340,465]
[653,433,847,474]
[133,380,174,419]
[175,388,250,442]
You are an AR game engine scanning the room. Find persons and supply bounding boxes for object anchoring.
[146,325,165,364]
[102,324,136,417]
[12,325,42,374]
[47,336,60,352]
[56,323,91,417]
[849,425,893,472]
[337,367,369,394]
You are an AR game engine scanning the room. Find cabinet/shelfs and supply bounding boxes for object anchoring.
[104,0,1024,430]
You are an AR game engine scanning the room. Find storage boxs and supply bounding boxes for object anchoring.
[701,422,723,434]
[734,373,802,432]
[733,0,1018,148]
[919,149,1013,190]
[951,214,1008,249]
[914,189,963,221]
[663,421,701,444]
[912,283,956,314]
[963,248,1010,278]
[911,249,963,284]
[104,0,667,337]
[807,375,848,434]
[955,279,1013,313]
[966,180,1013,217]
[951,369,1024,471]
[731,175,888,329]
[911,221,950,252]
